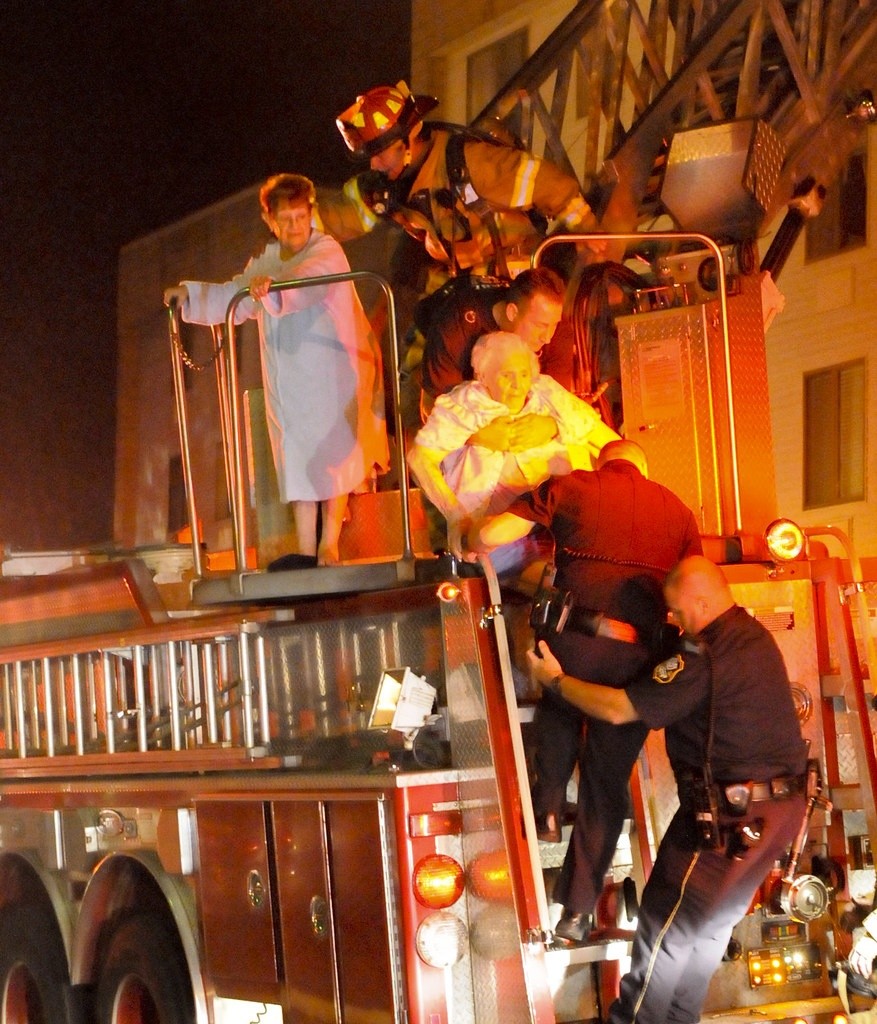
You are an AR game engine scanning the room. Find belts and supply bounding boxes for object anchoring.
[563,607,686,653]
[677,773,811,808]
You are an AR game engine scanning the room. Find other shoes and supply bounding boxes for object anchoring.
[268,552,318,572]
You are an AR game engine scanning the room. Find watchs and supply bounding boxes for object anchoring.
[548,674,568,695]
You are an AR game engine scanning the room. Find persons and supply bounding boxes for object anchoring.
[335,80,705,949]
[164,171,389,572]
[524,554,820,1023]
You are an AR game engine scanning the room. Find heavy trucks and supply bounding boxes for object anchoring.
[0,547,876,1023]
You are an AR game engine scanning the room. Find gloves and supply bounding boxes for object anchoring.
[848,927,877,981]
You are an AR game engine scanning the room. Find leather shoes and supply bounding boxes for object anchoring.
[534,809,562,843]
[555,908,591,943]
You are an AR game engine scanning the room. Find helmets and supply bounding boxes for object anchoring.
[335,78,441,164]
